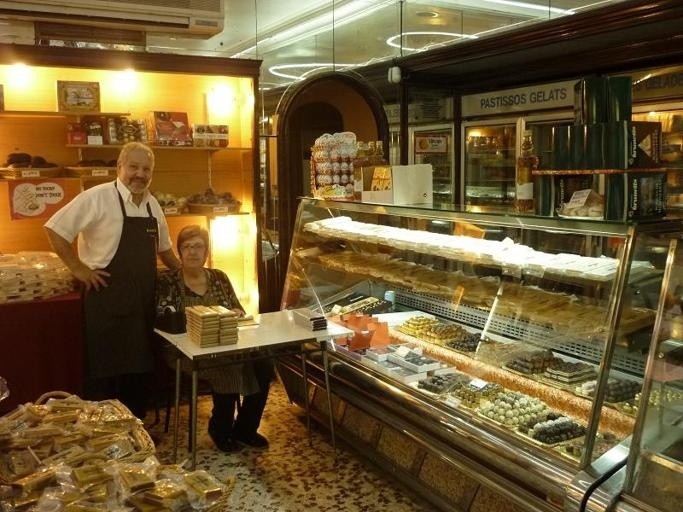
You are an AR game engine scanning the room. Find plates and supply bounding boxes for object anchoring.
[364,346,440,374]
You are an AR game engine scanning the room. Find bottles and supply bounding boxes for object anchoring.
[355,140,383,156]
[193,124,229,146]
[515,130,539,214]
[467,133,496,154]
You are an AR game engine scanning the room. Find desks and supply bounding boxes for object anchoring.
[153,307,356,470]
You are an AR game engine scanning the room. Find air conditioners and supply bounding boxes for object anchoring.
[0,0,226,40]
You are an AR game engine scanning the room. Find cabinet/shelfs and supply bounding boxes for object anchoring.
[0,108,250,219]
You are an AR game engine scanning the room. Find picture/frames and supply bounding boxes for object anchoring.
[55,80,101,115]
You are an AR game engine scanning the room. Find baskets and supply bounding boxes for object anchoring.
[34,391,157,476]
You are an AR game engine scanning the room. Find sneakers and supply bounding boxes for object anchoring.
[209,420,269,452]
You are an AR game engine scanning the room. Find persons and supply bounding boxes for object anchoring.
[42,142,182,419]
[154,224,270,454]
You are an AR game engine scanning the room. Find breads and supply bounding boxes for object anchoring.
[560,199,604,217]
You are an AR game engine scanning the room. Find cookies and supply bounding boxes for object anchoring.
[1,396,225,512]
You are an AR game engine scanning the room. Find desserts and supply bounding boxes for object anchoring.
[359,315,683,464]
